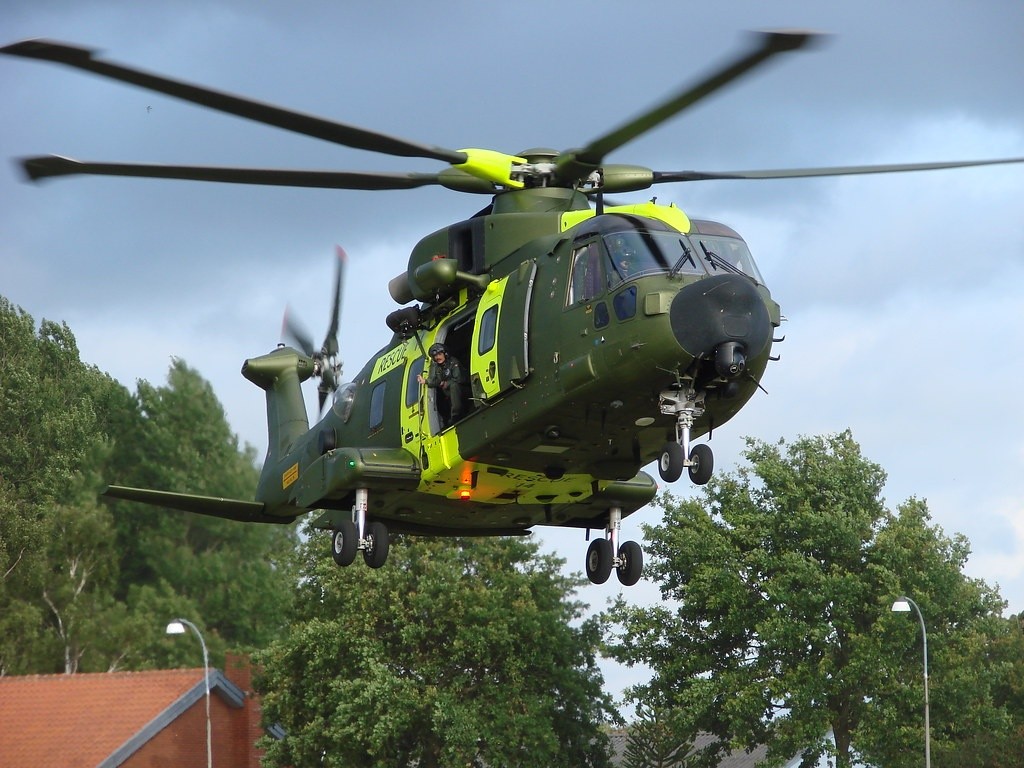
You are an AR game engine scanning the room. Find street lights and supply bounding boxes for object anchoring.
[891,594,930,768]
[167,616,213,768]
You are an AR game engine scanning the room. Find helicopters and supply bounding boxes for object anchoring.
[0,32,1024,586]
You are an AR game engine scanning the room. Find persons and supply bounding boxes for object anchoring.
[416,342,474,437]
[608,246,638,289]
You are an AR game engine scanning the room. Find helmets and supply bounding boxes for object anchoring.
[429,343,449,362]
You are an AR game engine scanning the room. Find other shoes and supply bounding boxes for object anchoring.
[448,417,462,425]
